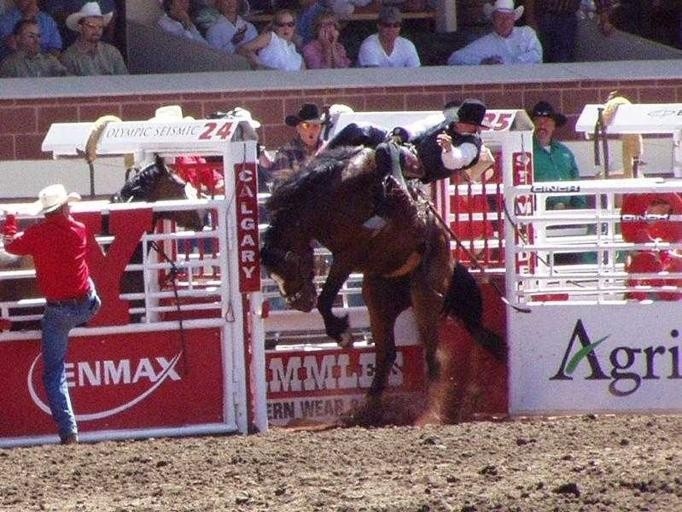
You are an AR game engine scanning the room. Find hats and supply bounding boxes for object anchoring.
[484,0,524,21]
[286,104,333,126]
[529,101,567,127]
[31,185,82,215]
[443,98,489,129]
[377,8,402,25]
[65,2,114,32]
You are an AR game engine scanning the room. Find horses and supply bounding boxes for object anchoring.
[1,151,210,333]
[258,147,506,426]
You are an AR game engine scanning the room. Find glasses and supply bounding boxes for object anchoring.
[279,21,294,29]
[83,21,104,30]
[301,123,320,128]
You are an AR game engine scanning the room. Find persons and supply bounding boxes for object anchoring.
[528,101,585,243]
[264,3,333,57]
[260,102,324,177]
[323,3,369,12]
[462,2,496,45]
[2,19,67,76]
[519,0,615,63]
[303,12,351,69]
[447,2,543,66]
[205,1,258,55]
[57,2,130,76]
[46,3,127,66]
[325,99,490,178]
[356,7,422,68]
[239,8,308,71]
[4,185,102,444]
[155,2,209,46]
[3,2,63,59]
[574,3,618,20]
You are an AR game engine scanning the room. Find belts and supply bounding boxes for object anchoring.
[47,288,93,307]
